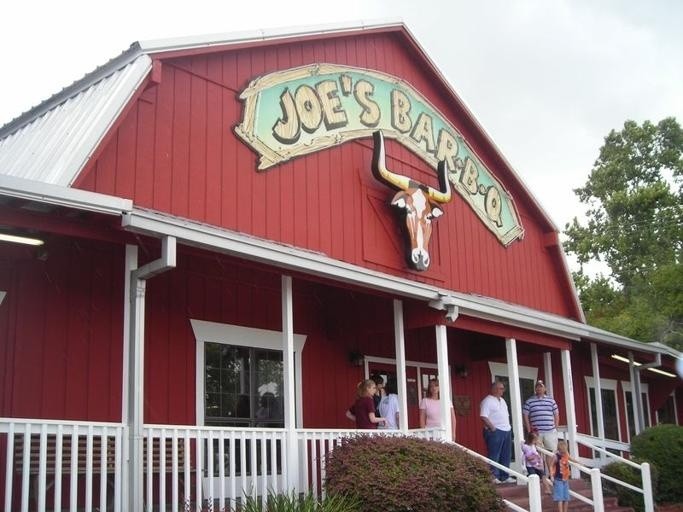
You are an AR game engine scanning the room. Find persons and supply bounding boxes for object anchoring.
[369,374,386,417]
[522,379,559,482]
[231,394,252,425]
[343,378,387,429]
[377,376,399,429]
[253,392,283,428]
[548,441,572,512]
[418,378,456,442]
[521,427,552,494]
[478,381,516,485]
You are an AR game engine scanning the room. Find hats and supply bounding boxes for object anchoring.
[535,380,545,386]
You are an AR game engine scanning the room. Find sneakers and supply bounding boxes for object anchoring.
[491,477,517,484]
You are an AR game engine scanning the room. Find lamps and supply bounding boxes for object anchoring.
[454,363,469,378]
[350,350,364,366]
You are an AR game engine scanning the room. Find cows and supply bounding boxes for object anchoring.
[373,128,453,273]
